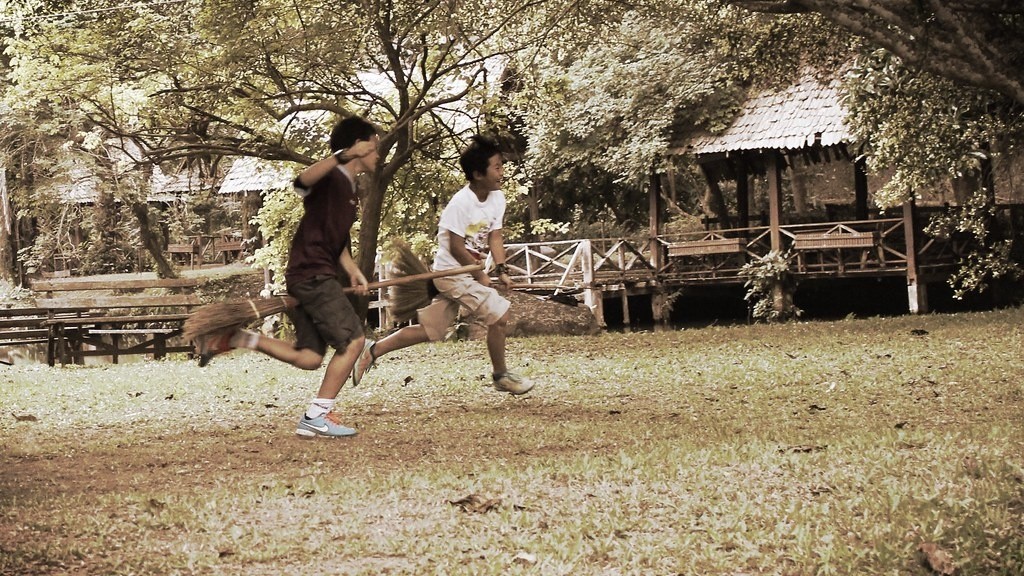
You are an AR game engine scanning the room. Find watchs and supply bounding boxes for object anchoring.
[496,263,508,269]
[333,148,353,166]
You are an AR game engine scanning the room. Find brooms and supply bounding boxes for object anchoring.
[180,260,486,349]
[385,240,587,323]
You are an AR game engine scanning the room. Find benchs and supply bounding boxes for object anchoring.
[31,278,202,369]
[0,308,94,365]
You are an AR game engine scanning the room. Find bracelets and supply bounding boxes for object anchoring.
[495,267,510,274]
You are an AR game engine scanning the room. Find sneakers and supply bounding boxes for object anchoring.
[492,370,536,395]
[295,410,357,437]
[353,338,380,387]
[198,328,239,368]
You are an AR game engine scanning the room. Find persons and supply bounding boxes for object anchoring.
[350,138,536,394]
[197,116,383,439]
